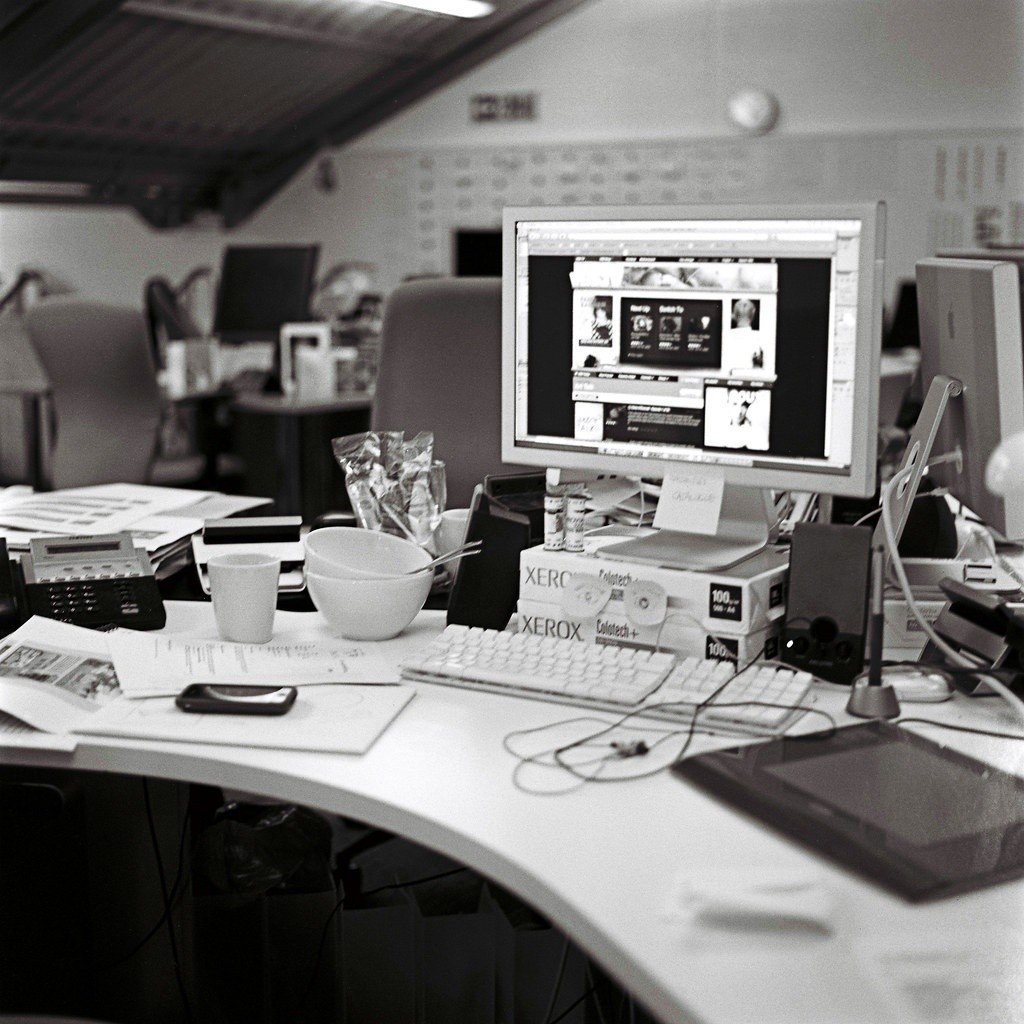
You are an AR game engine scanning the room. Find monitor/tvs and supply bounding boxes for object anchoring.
[214,243,320,352]
[499,201,886,571]
[866,255,1024,600]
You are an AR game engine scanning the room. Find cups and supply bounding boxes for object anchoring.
[208,552,281,645]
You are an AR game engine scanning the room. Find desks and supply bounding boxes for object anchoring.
[0,601,1024,1024]
[0,380,374,522]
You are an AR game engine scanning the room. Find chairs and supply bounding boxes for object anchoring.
[376,277,543,513]
[24,303,246,487]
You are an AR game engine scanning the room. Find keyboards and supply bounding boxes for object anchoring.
[398,624,819,737]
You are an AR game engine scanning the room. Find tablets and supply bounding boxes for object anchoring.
[694,719,1024,885]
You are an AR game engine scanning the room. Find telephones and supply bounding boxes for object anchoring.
[0,531,166,640]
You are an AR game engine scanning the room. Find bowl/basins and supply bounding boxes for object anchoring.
[304,525,433,642]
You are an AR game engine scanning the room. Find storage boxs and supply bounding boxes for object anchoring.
[883,602,1024,648]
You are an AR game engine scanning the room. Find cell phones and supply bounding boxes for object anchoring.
[176,681,297,715]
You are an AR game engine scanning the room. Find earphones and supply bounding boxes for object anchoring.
[612,740,647,757]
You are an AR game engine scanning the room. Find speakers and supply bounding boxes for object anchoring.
[448,484,532,631]
[779,521,873,685]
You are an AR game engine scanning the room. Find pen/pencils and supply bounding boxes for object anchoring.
[869,543,885,688]
